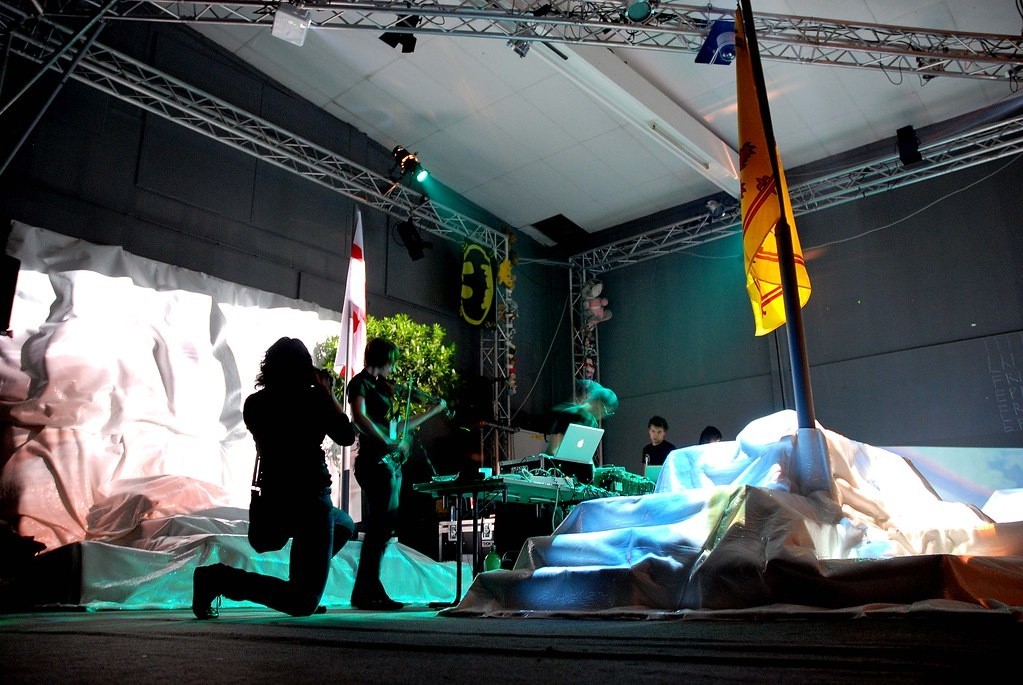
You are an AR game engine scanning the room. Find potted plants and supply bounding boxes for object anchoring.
[314,313,458,456]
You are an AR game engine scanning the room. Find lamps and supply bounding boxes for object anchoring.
[647,120,710,169]
[706,200,725,219]
[392,217,426,263]
[379,3,421,53]
[1005,65,1023,79]
[717,32,736,62]
[385,145,431,187]
[897,124,922,168]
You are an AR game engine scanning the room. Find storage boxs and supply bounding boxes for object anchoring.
[438,518,495,562]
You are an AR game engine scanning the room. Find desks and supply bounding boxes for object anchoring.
[413,477,599,609]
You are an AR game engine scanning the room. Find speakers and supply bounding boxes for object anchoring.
[897,125,922,166]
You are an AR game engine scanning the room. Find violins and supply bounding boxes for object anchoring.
[376,376,456,422]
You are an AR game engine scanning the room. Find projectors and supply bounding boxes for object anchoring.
[271,3,313,46]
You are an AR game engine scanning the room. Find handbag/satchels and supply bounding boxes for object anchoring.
[248,490,290,554]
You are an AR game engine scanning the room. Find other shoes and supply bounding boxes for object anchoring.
[315,606,326,614]
[350,577,405,611]
[192,563,226,621]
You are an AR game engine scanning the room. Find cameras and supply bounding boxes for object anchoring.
[312,366,324,386]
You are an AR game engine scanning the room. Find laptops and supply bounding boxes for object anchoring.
[546,424,604,464]
[645,465,664,483]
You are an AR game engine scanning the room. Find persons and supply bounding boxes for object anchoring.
[346,338,447,610]
[699,426,723,446]
[640,416,677,467]
[545,379,619,533]
[192,337,356,621]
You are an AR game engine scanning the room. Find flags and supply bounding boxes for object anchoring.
[730,5,811,338]
[333,212,367,420]
